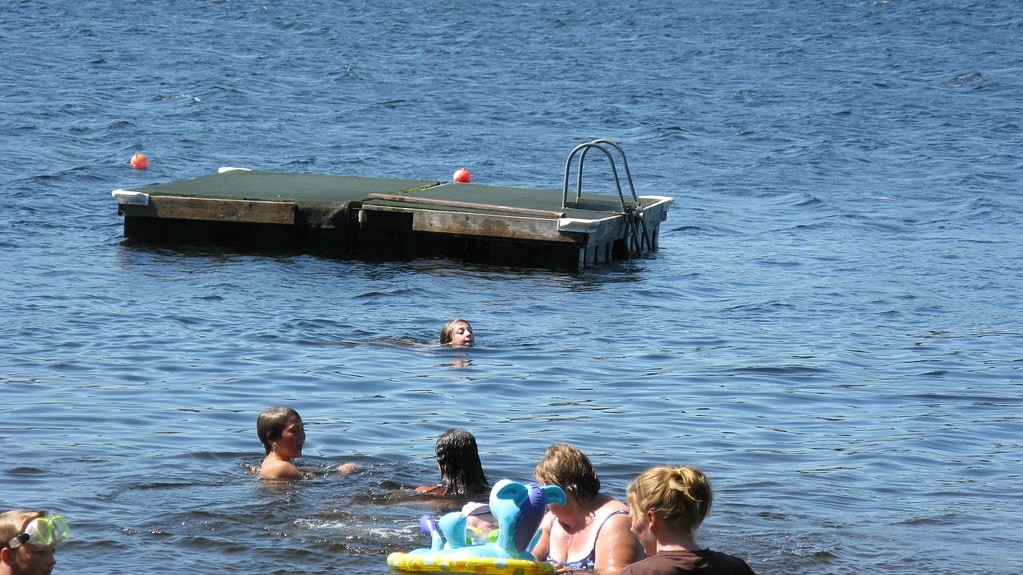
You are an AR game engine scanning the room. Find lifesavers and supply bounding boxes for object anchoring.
[386,478,567,575]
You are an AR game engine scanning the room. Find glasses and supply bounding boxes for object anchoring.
[0,515,71,551]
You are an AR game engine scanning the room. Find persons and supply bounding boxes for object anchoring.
[0,508,69,575]
[531,442,636,575]
[387,428,491,510]
[252,406,358,493]
[440,319,475,347]
[622,466,758,575]
